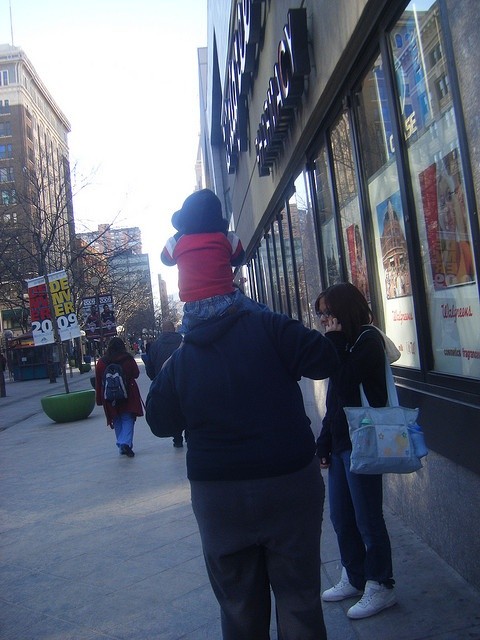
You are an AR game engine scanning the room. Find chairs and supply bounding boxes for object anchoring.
[346,580,397,620]
[321,567,364,601]
[121,444,134,458]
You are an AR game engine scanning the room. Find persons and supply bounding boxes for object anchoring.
[145,282,341,640]
[146,322,184,448]
[100,304,115,325]
[314,283,398,621]
[160,189,270,335]
[85,305,99,328]
[95,336,144,459]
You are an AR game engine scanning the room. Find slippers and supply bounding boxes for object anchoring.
[101,356,129,407]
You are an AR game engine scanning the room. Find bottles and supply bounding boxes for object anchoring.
[172,188,228,234]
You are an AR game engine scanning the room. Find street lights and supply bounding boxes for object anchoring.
[343,328,428,474]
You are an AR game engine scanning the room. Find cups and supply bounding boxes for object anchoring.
[316,311,332,318]
